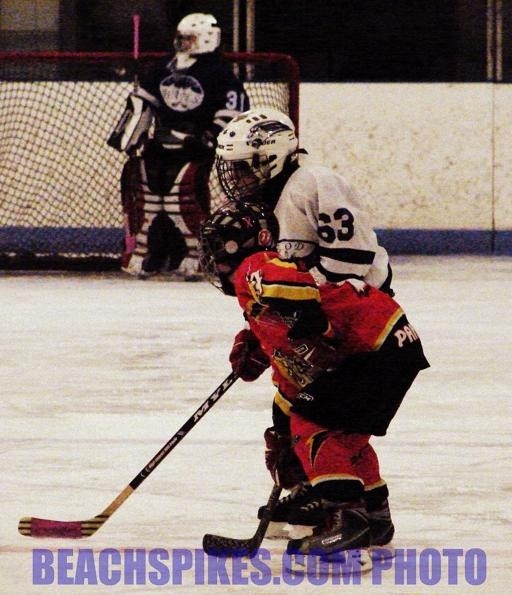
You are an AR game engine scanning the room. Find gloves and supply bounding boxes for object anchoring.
[284,336,338,370]
[264,427,304,488]
[231,329,269,383]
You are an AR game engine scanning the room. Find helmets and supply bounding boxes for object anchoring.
[199,202,280,296]
[215,108,298,201]
[173,13,221,54]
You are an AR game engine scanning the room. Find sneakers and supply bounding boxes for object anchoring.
[258,483,395,555]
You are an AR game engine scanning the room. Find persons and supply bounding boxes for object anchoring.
[211,103,396,547]
[118,10,251,281]
[195,198,430,577]
